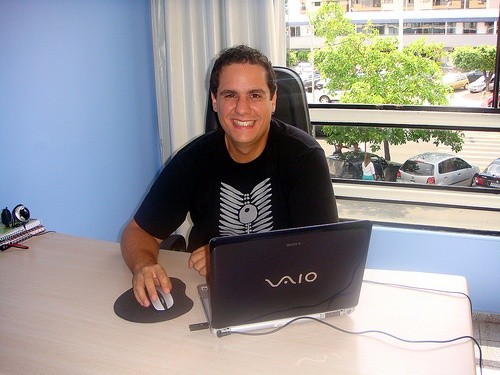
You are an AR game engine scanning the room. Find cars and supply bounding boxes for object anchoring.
[326,152,403,182]
[442,73,469,90]
[294,63,351,103]
[468,74,500,92]
[395,152,480,188]
[469,158,500,188]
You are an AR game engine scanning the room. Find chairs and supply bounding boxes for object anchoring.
[158,66,313,253]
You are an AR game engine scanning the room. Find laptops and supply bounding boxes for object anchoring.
[196,220,373,339]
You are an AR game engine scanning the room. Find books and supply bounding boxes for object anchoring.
[0,218,46,251]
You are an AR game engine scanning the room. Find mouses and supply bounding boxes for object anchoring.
[145,285,175,312]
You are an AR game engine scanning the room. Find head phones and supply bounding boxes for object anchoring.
[1,203,30,228]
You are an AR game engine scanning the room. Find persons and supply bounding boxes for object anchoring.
[362,152,377,181]
[120,44,339,308]
[348,142,362,152]
[333,146,342,155]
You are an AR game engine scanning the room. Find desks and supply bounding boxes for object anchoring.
[0,231,477,375]
